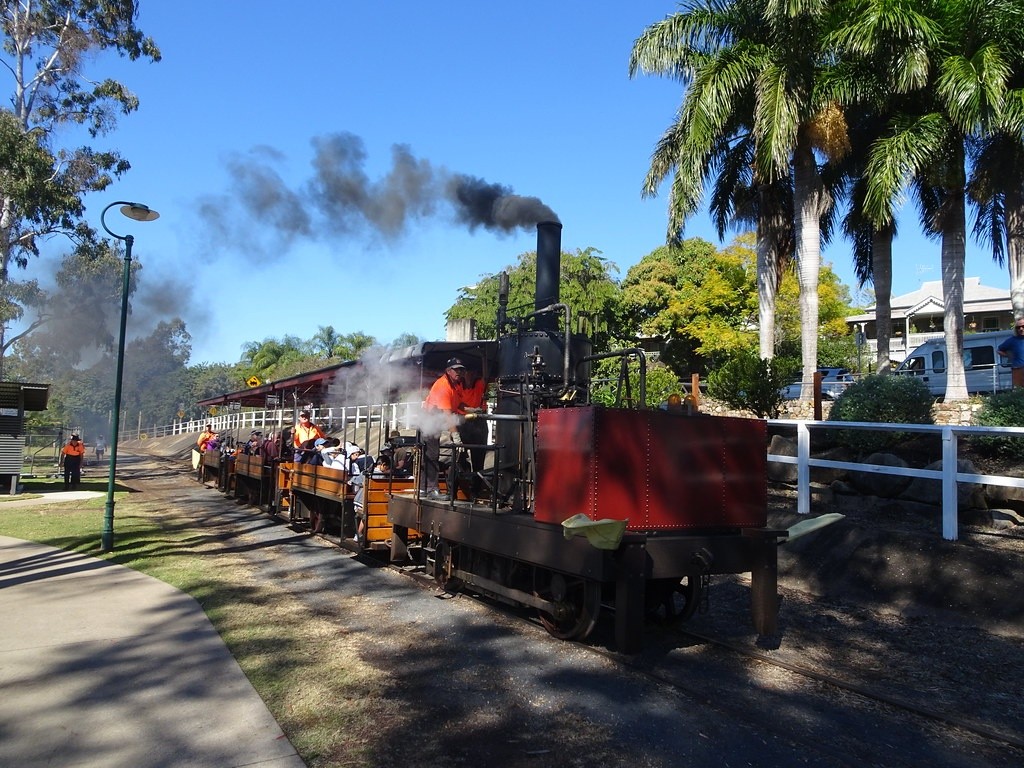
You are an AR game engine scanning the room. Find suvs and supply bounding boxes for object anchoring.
[777,367,855,402]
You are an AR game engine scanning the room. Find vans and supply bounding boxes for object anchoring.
[893,330,1014,396]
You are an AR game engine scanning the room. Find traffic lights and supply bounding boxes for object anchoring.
[856,333,863,345]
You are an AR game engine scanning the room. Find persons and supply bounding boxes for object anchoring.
[58,433,85,490]
[909,358,922,375]
[996,317,1024,391]
[456,367,490,499]
[418,357,465,500]
[197,424,214,452]
[92,435,107,461]
[206,409,407,542]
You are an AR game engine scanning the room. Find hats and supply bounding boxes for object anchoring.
[69,433,82,441]
[314,437,330,446]
[247,430,262,437]
[446,358,466,369]
[346,446,360,458]
[344,442,353,450]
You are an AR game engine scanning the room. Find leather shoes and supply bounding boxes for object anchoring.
[415,487,428,499]
[427,489,449,501]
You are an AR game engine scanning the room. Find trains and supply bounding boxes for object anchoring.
[191,221,790,647]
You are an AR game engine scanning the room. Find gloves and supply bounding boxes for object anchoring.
[450,431,464,452]
[464,406,482,413]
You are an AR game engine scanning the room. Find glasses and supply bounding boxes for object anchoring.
[300,413,310,418]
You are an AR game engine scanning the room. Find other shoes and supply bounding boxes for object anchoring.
[352,534,364,541]
[444,491,457,500]
[466,495,479,502]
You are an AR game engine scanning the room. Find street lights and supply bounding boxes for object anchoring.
[101,201,159,551]
